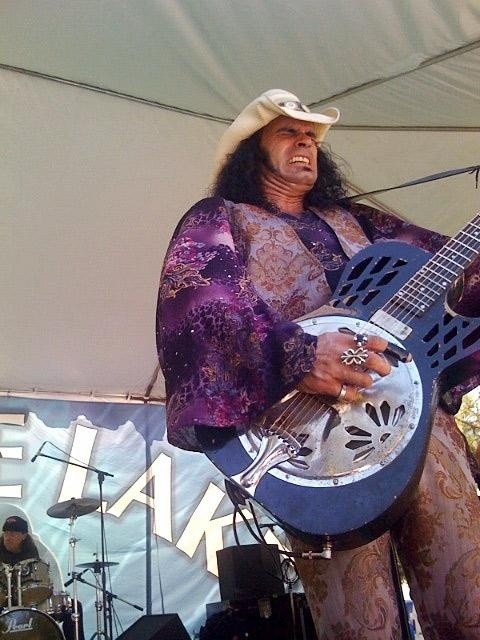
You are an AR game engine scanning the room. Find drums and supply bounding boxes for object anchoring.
[0,606,65,639]
[0,562,8,605]
[34,590,72,622]
[11,558,54,605]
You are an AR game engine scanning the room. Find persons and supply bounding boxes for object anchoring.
[155,87,480,640]
[1,516,86,640]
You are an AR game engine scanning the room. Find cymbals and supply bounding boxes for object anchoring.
[74,562,119,568]
[47,498,100,518]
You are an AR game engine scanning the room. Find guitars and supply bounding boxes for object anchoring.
[195,209,480,553]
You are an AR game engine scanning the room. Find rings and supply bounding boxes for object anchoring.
[338,346,368,368]
[352,332,368,346]
[335,386,347,401]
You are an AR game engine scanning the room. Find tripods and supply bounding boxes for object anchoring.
[90,573,109,640]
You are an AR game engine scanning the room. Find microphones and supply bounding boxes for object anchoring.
[64,569,88,587]
[31,441,46,462]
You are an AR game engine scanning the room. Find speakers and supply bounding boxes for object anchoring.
[216,544,285,602]
[114,613,192,640]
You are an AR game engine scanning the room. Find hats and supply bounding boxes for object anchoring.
[2,516,28,534]
[208,87,342,197]
